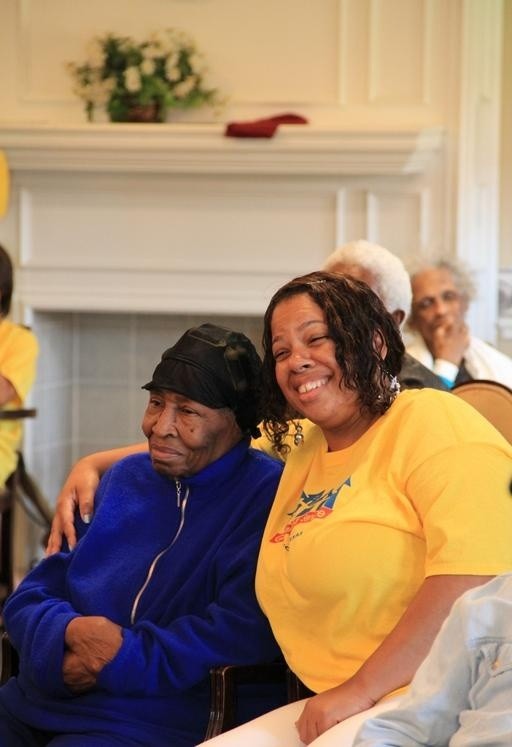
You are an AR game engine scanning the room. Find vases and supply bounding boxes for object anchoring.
[114,103,160,123]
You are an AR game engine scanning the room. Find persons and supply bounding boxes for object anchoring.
[3,241,510,747]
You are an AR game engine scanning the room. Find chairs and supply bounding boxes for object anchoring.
[198,657,292,744]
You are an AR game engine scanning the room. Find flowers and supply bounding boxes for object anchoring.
[66,24,216,120]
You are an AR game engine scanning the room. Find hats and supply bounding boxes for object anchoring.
[141,323,263,408]
